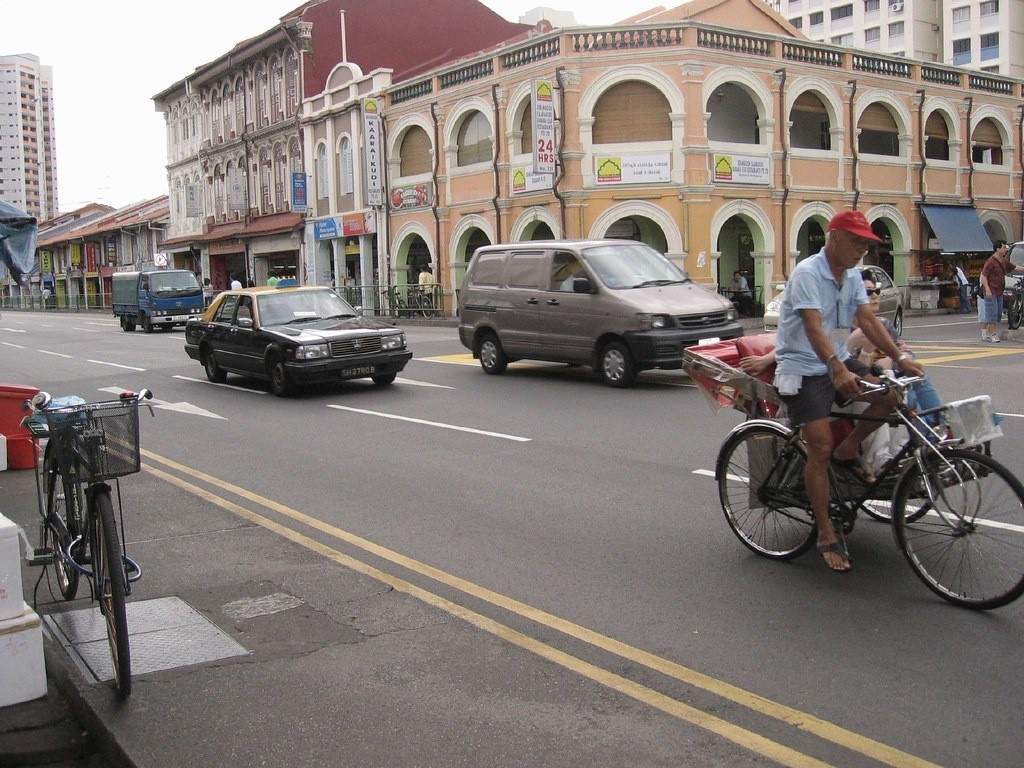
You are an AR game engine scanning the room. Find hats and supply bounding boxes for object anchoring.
[826,211,882,242]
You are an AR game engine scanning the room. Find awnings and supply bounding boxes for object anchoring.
[920,204,994,265]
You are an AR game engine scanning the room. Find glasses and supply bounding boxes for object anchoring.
[866,288,880,296]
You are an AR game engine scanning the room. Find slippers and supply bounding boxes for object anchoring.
[815,540,855,573]
[830,455,872,488]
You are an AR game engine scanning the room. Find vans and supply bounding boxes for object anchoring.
[458,238,744,388]
[1002,241,1024,299]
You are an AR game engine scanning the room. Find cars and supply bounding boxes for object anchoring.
[764,265,905,338]
[184,277,413,397]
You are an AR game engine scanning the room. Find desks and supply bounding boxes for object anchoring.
[902,281,955,286]
[724,289,760,318]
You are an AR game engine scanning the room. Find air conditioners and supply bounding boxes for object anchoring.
[892,3,901,11]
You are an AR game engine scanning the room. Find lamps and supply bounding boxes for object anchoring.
[716,89,724,101]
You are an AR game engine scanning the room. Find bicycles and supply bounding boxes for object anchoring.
[406,287,433,320]
[21,388,154,700]
[1007,277,1024,329]
[381,285,410,319]
[681,332,1024,610]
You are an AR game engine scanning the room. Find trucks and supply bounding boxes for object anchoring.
[112,269,210,332]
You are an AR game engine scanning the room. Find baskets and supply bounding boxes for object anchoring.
[46,397,141,484]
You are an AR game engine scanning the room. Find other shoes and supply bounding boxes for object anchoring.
[874,453,895,473]
[989,332,1000,343]
[981,328,989,341]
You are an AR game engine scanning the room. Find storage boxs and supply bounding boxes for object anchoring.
[907,286,962,310]
[0,433,47,707]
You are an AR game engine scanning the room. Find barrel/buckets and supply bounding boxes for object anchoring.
[5,433,43,470]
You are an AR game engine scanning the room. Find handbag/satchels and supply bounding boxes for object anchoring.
[977,284,985,300]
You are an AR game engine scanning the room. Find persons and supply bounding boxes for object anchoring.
[848,265,946,445]
[949,264,973,313]
[228,272,243,299]
[772,211,923,574]
[202,280,214,308]
[417,264,435,316]
[267,271,279,287]
[727,271,753,317]
[976,239,1024,343]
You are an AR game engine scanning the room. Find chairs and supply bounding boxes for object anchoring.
[719,286,764,317]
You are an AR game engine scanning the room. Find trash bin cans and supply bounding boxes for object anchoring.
[0,384,40,435]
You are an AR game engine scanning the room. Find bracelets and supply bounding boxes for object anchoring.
[895,353,910,364]
